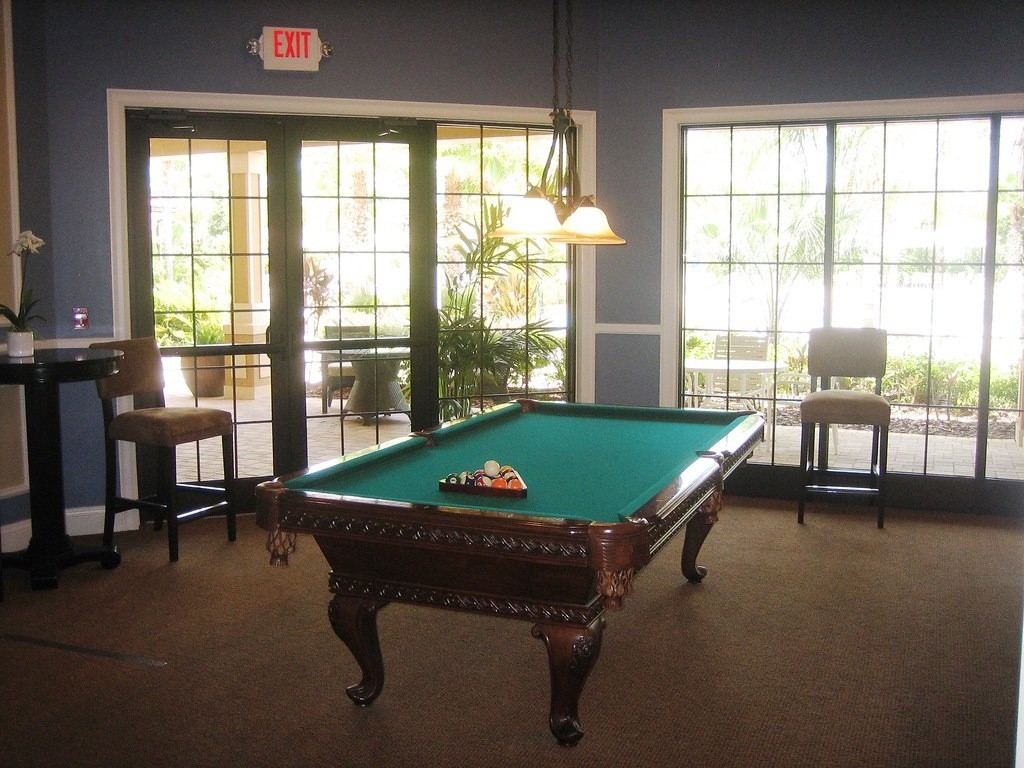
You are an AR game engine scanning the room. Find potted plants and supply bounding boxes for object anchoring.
[153,307,226,398]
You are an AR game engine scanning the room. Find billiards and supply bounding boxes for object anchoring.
[445,459,522,489]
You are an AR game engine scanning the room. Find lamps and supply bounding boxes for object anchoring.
[488,1,625,246]
[246,27,334,72]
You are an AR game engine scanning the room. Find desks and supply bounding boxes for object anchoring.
[685,359,790,418]
[317,346,410,424]
[0,348,125,591]
[255,399,766,748]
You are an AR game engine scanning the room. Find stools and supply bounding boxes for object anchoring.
[90,337,237,561]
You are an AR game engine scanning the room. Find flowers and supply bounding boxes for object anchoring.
[0,230,47,342]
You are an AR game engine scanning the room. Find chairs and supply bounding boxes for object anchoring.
[798,327,888,528]
[698,335,839,455]
[322,326,370,414]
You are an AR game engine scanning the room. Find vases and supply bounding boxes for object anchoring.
[6,332,34,357]
